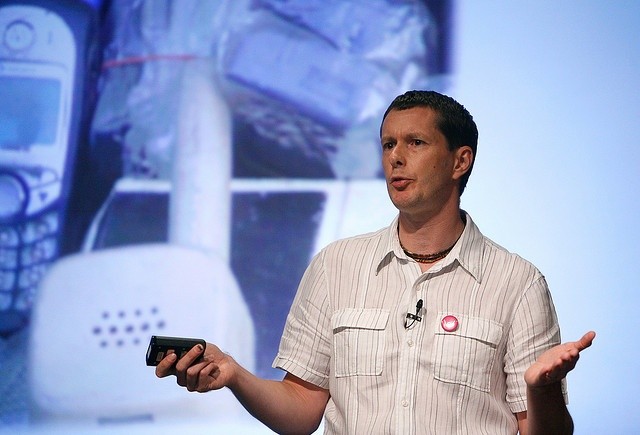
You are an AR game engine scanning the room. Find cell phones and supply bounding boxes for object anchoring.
[146,336,205,367]
[0,5,84,336]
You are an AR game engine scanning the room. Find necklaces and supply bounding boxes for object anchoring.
[398,236,460,263]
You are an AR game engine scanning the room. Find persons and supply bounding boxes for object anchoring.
[155,89,596,434]
